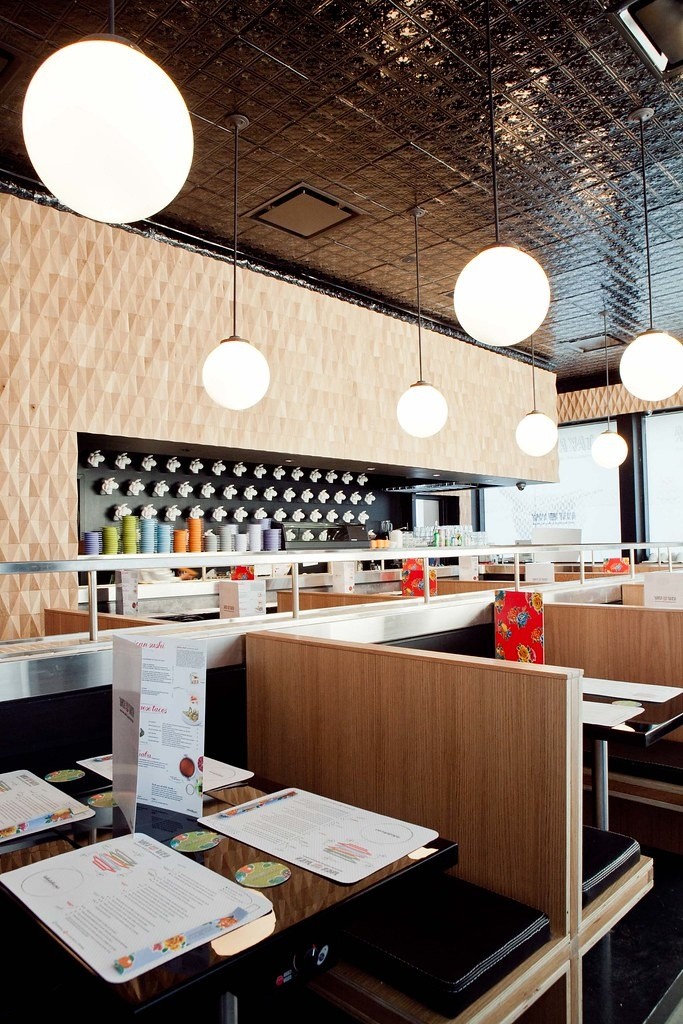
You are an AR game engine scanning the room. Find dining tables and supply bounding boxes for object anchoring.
[1,753,460,1024]
[583,679,683,836]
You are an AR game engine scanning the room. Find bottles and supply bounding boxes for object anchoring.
[433,530,439,546]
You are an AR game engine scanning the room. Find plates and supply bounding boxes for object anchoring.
[82,516,282,555]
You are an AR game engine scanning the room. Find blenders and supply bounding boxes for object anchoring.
[380,520,392,540]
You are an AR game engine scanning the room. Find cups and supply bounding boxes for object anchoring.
[370,539,390,548]
[413,525,489,546]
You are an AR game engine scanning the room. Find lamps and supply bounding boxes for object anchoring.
[453,10,551,348]
[517,333,558,458]
[618,108,683,404]
[20,0,194,224]
[586,309,628,467]
[204,116,269,412]
[395,206,448,440]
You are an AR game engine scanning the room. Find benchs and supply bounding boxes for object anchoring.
[245,631,581,1024]
[583,738,683,815]
[582,824,654,962]
